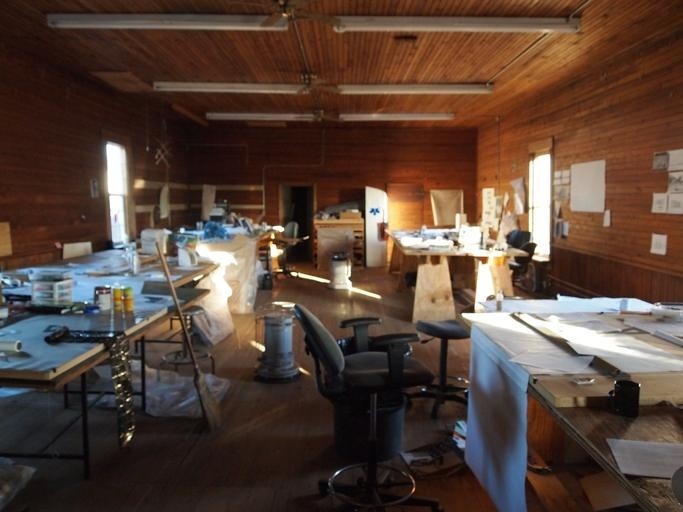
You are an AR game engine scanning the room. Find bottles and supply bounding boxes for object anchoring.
[496,290,504,313]
[112,213,121,243]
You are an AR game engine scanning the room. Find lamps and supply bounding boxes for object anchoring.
[335,83,494,95]
[151,80,309,97]
[339,113,456,122]
[332,14,582,35]
[205,112,318,122]
[45,13,292,33]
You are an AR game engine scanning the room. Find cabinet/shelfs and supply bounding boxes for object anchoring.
[313,215,365,265]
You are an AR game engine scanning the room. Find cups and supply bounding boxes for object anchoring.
[608,378,640,419]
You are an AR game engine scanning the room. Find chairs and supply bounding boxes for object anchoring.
[63,241,92,259]
[506,231,530,249]
[274,221,299,279]
[293,304,434,512]
[509,242,536,286]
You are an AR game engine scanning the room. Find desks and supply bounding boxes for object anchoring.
[462,308,683,511]
[383,225,528,323]
[183,224,273,290]
[0,262,221,287]
[0,285,210,474]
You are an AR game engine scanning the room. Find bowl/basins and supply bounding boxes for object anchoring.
[652,302,682,324]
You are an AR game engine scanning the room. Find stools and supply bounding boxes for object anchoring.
[406,318,468,418]
[160,303,215,378]
